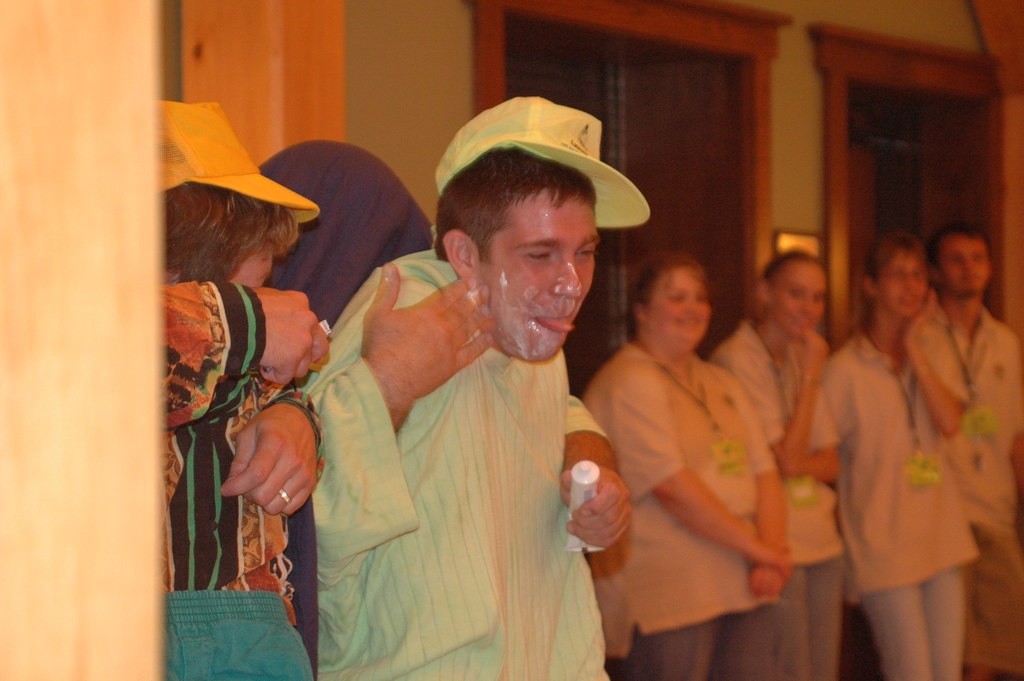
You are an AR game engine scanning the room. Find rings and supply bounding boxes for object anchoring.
[278,489,294,506]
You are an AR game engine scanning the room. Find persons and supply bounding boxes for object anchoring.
[578,225,1024,681]
[295,96,651,681]
[160,100,332,681]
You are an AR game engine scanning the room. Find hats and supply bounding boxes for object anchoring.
[159,101,321,224]
[434,96,650,227]
